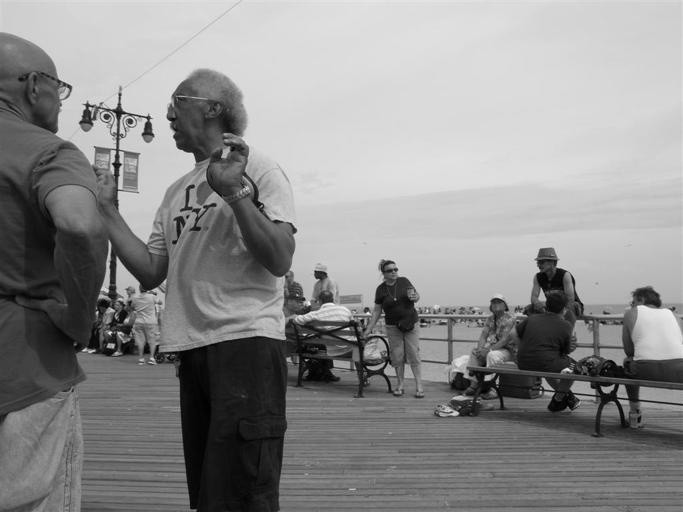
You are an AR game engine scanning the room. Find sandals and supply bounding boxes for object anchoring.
[460,383,478,396]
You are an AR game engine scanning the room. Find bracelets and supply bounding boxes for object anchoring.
[221,186,252,205]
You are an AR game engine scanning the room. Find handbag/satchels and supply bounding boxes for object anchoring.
[350,339,382,362]
[396,316,414,332]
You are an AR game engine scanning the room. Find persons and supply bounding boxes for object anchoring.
[83,284,162,364]
[517,247,585,411]
[464,295,521,399]
[0,31,108,512]
[284,259,425,398]
[621,286,683,429]
[99,69,299,512]
[351,304,528,327]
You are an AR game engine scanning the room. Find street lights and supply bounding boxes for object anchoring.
[79,85,155,301]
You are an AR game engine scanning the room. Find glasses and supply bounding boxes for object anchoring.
[17,70,73,102]
[167,94,221,109]
[384,267,398,273]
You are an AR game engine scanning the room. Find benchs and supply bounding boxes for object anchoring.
[467,365,683,438]
[284,318,394,399]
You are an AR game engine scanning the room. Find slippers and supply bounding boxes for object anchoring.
[415,388,424,398]
[393,387,405,396]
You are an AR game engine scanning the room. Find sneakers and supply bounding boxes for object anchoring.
[303,373,341,382]
[82,348,156,365]
[627,411,644,429]
[548,395,568,412]
[566,389,581,411]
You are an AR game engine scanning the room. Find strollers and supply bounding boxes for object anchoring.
[154,305,174,364]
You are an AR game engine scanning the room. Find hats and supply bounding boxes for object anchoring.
[311,263,328,274]
[534,247,559,261]
[489,294,510,313]
